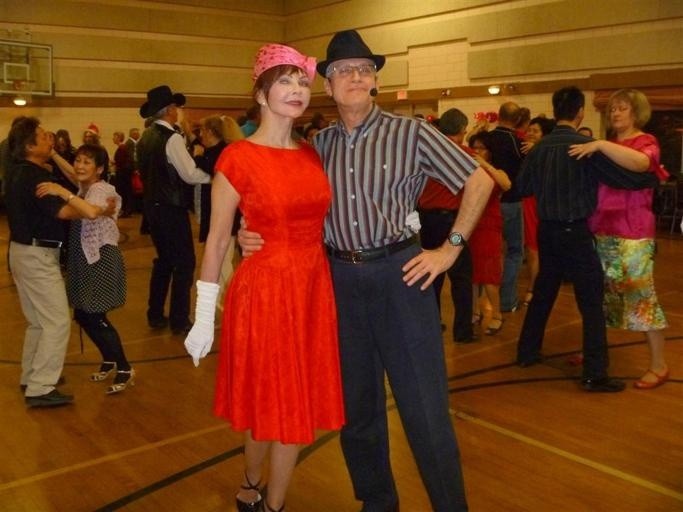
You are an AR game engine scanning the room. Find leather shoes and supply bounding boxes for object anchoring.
[517,353,543,367]
[454,335,481,343]
[172,321,192,336]
[148,316,168,328]
[569,352,583,365]
[25,390,73,406]
[21,378,64,391]
[582,376,626,392]
[633,364,670,390]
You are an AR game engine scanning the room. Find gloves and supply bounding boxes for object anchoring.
[184,280,220,368]
[405,211,421,233]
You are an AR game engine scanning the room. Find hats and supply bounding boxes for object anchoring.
[316,30,385,77]
[252,44,316,85]
[140,85,185,118]
[85,122,98,135]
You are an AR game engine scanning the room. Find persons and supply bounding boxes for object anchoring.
[390,102,556,343]
[0,116,141,406]
[184,45,348,511]
[172,112,242,327]
[236,103,262,137]
[136,84,211,334]
[289,112,330,144]
[515,83,671,392]
[239,28,495,511]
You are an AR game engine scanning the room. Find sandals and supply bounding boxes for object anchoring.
[522,291,534,307]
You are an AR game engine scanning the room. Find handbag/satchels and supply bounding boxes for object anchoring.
[561,219,597,257]
[132,174,144,195]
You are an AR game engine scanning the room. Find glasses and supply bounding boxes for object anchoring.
[471,145,488,151]
[327,64,377,76]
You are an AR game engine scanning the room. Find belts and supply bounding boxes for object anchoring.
[324,234,418,264]
[12,240,59,247]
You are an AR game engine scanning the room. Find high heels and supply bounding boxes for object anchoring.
[484,315,504,335]
[471,311,483,325]
[236,470,263,512]
[106,368,136,394]
[89,362,117,381]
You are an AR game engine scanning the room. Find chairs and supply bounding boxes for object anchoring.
[658,181,683,233]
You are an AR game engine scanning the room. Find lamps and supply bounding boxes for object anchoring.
[13,95,28,106]
[488,85,500,95]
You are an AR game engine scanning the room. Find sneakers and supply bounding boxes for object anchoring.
[500,299,519,312]
